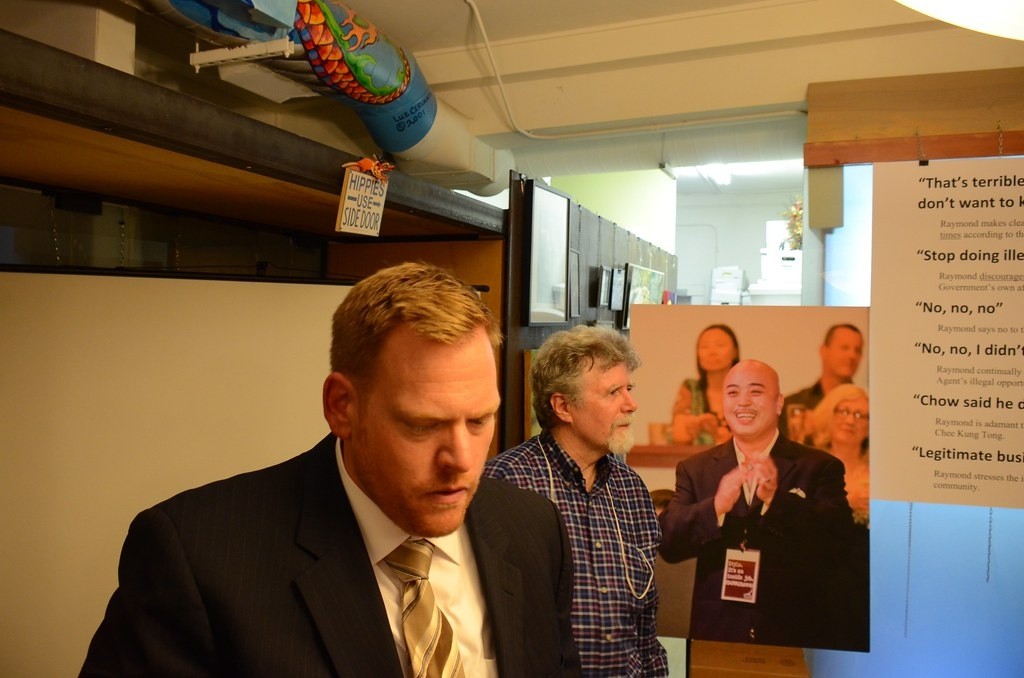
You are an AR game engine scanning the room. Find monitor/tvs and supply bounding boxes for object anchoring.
[620,263,665,329]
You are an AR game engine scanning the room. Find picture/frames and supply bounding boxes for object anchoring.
[610,268,626,310]
[598,265,612,307]
[520,180,573,326]
[620,262,665,331]
[570,248,581,318]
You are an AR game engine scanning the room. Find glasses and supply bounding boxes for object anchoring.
[835,408,868,421]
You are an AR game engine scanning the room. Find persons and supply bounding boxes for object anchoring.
[479,325,670,678]
[79,262,581,678]
[656,324,870,651]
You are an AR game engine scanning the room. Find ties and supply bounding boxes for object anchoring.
[384,536,467,678]
